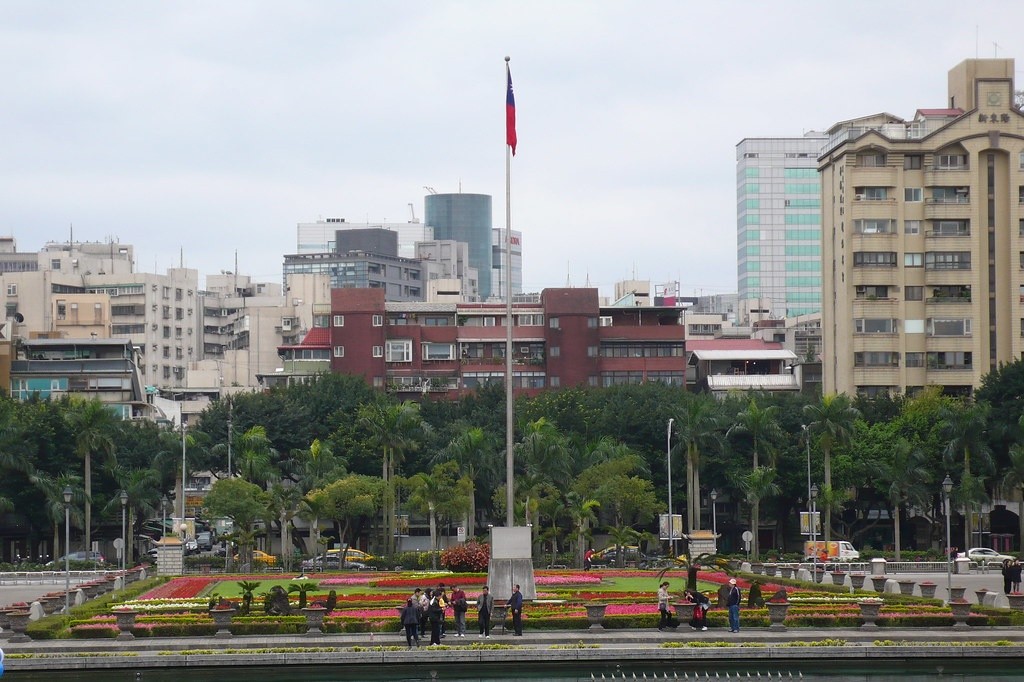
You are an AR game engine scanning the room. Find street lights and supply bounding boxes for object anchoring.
[61,483,74,614]
[667,417,677,560]
[810,482,820,583]
[161,493,169,578]
[181,422,188,540]
[942,474,954,601]
[120,489,128,590]
[709,488,719,554]
[801,423,812,543]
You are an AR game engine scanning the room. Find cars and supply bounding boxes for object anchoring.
[46,550,106,568]
[592,546,649,569]
[236,549,277,569]
[327,548,376,563]
[214,545,240,558]
[956,547,1018,566]
[301,554,366,571]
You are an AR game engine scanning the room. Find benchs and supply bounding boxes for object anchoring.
[357,567,378,571]
[264,567,283,573]
[302,567,323,572]
[546,565,567,569]
[591,564,607,569]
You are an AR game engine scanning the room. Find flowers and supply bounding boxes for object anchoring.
[769,596,786,603]
[810,568,1022,595]
[676,599,692,604]
[862,599,877,604]
[213,602,232,610]
[953,599,968,604]
[307,602,323,608]
[1,565,151,615]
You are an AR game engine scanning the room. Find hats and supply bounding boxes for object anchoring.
[729,579,736,585]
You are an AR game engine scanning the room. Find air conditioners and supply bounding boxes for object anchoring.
[855,194,865,201]
[461,342,469,349]
[521,347,529,353]
[174,368,179,373]
[955,187,968,193]
[856,285,866,293]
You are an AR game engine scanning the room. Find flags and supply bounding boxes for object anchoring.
[506,68,517,156]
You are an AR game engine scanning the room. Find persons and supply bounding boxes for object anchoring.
[1002,559,1022,594]
[477,586,494,638]
[584,549,595,571]
[950,548,957,561]
[15,554,51,564]
[401,583,466,648]
[658,582,673,632]
[685,590,711,630]
[504,585,523,635]
[727,579,741,632]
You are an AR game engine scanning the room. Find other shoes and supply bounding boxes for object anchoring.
[728,629,739,633]
[692,627,696,630]
[702,626,707,631]
[422,634,428,637]
[513,633,522,636]
[461,634,465,638]
[479,633,483,639]
[454,634,460,637]
[485,635,490,639]
[431,642,442,646]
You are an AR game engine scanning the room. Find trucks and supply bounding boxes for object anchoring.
[173,517,200,556]
[804,540,862,563]
[197,531,214,551]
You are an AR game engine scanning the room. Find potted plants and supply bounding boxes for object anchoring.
[1,639,1024,670]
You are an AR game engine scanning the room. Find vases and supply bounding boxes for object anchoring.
[583,604,607,629]
[0,567,149,637]
[671,603,697,627]
[300,608,327,633]
[751,565,764,576]
[810,571,1024,610]
[210,609,236,633]
[948,602,973,627]
[857,602,883,627]
[726,561,739,571]
[764,565,778,577]
[490,605,511,629]
[765,603,790,627]
[779,567,792,579]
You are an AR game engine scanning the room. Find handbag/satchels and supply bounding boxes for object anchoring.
[693,605,702,620]
[660,603,666,610]
